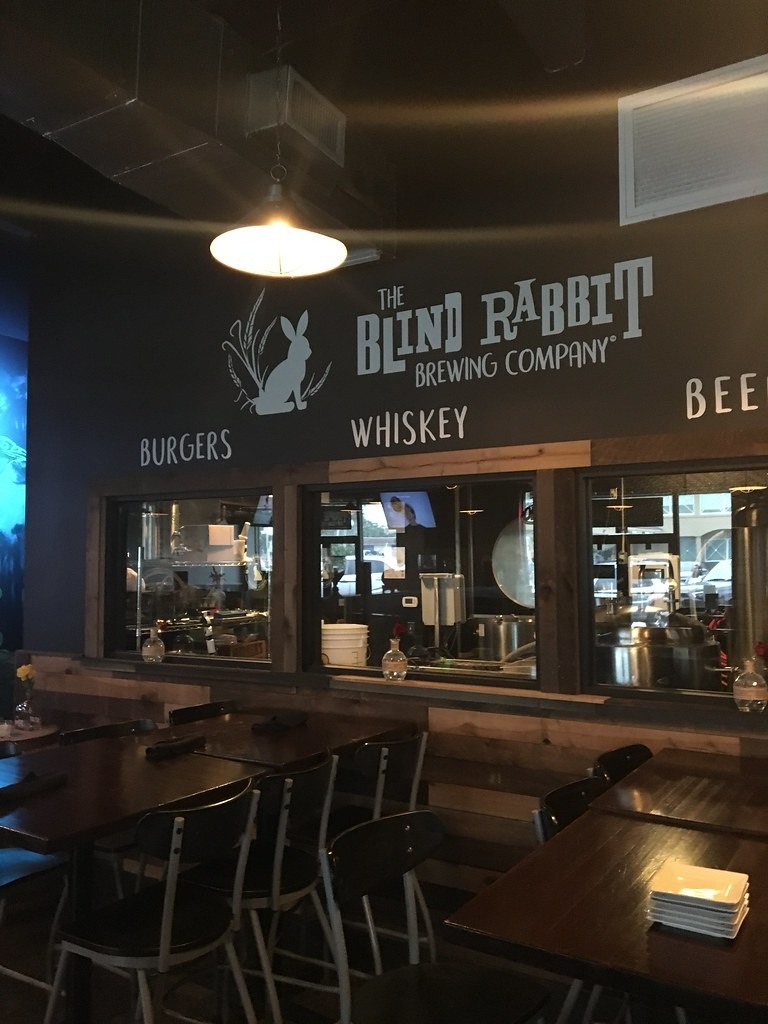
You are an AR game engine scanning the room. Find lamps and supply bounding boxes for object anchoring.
[210,2,350,278]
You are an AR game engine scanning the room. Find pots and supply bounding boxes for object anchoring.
[476,615,536,662]
[600,625,720,688]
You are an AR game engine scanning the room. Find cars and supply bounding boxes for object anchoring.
[674,558,734,613]
[440,613,537,673]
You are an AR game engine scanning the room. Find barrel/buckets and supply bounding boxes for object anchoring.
[321,620,371,666]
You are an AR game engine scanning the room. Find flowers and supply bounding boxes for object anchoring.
[751,642,766,661]
[392,624,409,641]
[15,664,35,692]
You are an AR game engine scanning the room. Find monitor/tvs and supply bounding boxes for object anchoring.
[379,491,437,528]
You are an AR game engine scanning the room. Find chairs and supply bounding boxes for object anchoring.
[43,731,689,1023]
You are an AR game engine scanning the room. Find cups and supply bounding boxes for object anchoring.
[0,720,13,737]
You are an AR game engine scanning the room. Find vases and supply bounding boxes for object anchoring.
[13,692,42,731]
[141,627,164,664]
[731,661,768,712]
[382,637,406,682]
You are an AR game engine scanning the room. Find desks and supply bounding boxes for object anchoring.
[1,669,417,1024]
[443,747,768,1024]
[125,618,268,651]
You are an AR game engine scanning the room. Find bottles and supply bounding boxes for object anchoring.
[606,590,624,615]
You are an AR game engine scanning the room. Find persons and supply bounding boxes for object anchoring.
[387,496,425,530]
